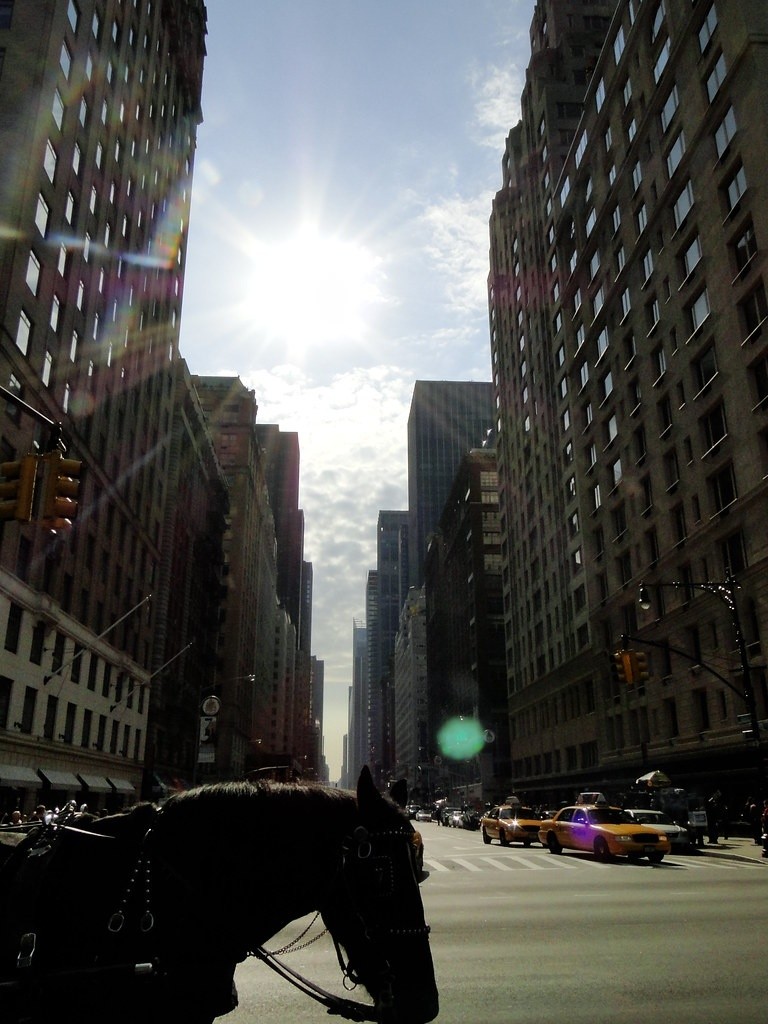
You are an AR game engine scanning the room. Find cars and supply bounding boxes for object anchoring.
[617,807,691,845]
[406,803,466,828]
[480,795,543,847]
[536,791,671,865]
[541,810,558,819]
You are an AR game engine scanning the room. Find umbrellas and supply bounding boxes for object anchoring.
[636,768,670,787]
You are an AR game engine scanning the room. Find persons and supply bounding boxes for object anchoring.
[435,801,576,828]
[80,804,108,818]
[0,805,46,827]
[615,791,768,858]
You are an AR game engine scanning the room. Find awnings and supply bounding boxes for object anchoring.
[108,777,135,793]
[39,768,82,790]
[0,764,43,788]
[78,773,112,793]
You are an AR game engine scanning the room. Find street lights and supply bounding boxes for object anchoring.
[637,568,765,845]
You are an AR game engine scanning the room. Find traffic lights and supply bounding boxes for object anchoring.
[630,651,650,682]
[38,449,84,536]
[0,453,40,522]
[608,649,635,683]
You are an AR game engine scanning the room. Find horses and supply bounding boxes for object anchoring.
[0,762,440,1024]
